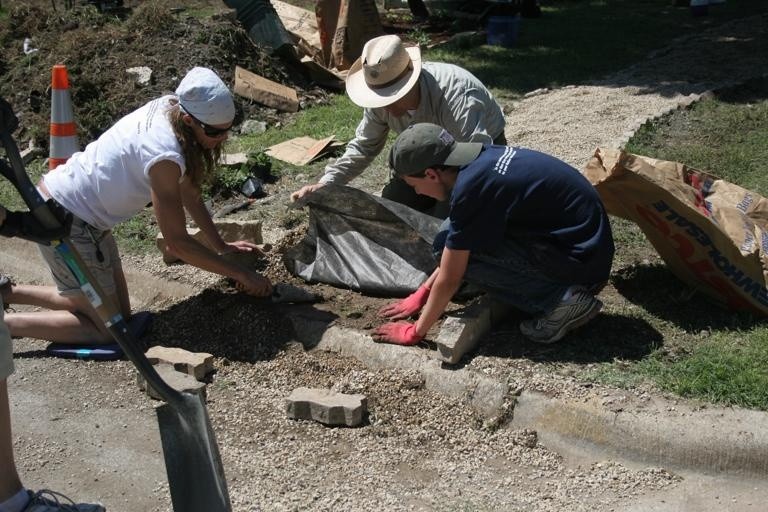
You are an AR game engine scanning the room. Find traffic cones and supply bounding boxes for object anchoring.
[49,64,80,172]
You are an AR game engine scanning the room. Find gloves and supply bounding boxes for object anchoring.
[369,320,427,347]
[378,284,432,322]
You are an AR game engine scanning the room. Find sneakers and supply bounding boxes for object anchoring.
[519,287,603,345]
[21,488,106,512]
[0,274,13,310]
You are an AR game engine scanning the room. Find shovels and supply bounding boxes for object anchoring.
[0,132,232,512]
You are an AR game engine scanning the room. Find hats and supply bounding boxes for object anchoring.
[388,122,484,177]
[345,35,424,109]
[174,66,236,126]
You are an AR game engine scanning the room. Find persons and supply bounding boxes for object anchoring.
[0,64,276,348]
[1,292,108,512]
[369,122,616,348]
[289,32,508,212]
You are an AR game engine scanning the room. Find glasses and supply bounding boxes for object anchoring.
[195,119,232,138]
